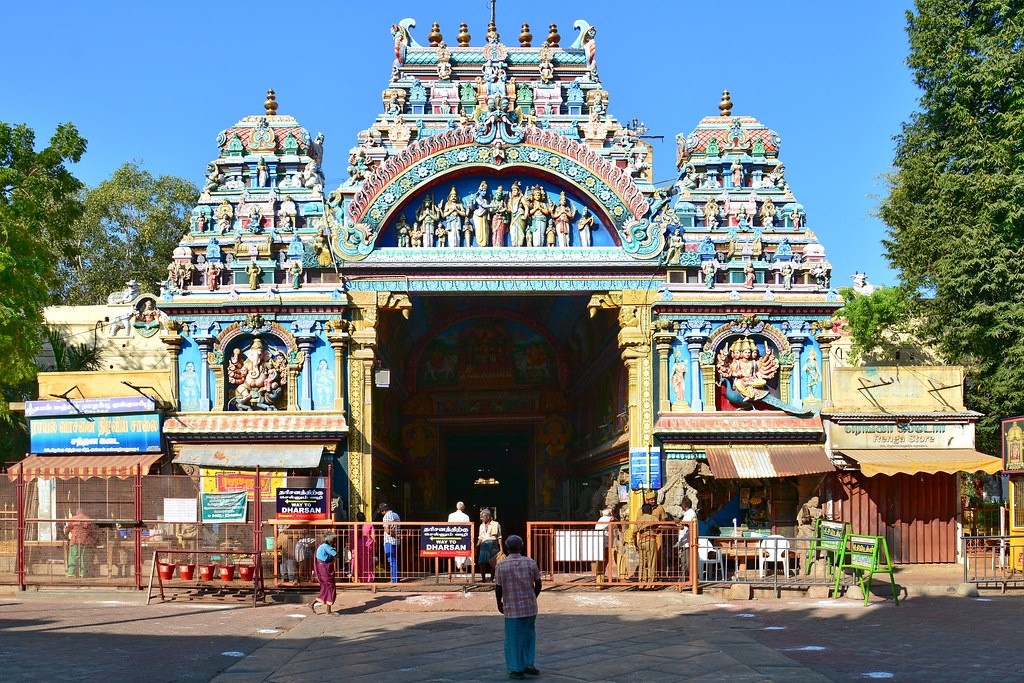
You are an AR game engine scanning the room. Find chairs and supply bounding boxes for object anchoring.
[757,533,791,580]
[695,538,726,581]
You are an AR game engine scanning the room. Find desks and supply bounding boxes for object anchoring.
[22,540,173,582]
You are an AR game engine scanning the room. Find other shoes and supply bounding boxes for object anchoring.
[509,671,524,679]
[524,667,539,674]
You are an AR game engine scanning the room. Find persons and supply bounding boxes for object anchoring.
[396,181,595,247]
[307,531,341,617]
[344,503,402,584]
[593,492,721,589]
[493,535,542,679]
[64,507,97,578]
[661,158,825,290]
[476,509,503,583]
[138,156,330,324]
[448,501,476,573]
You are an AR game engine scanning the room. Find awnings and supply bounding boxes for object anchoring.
[170,444,324,468]
[7,454,165,482]
[839,448,1003,478]
[702,445,835,479]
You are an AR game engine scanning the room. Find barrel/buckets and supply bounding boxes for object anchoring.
[239,564,255,581]
[265,536,274,549]
[178,564,194,580]
[198,564,215,581]
[160,563,175,579]
[219,565,235,582]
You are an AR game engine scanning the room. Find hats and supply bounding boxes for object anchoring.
[646,492,655,499]
